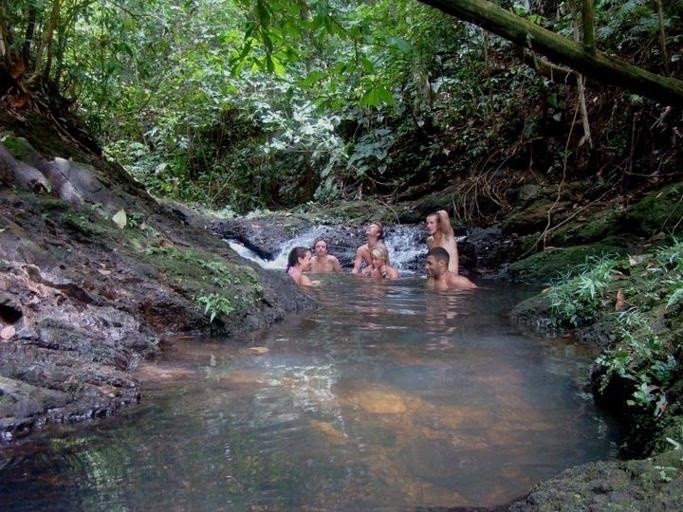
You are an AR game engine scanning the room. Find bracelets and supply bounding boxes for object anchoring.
[381,271,387,279]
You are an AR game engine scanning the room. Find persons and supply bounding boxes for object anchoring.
[425,210,459,275]
[302,240,341,274]
[360,245,398,280]
[351,221,390,276]
[424,247,479,291]
[286,246,320,288]
